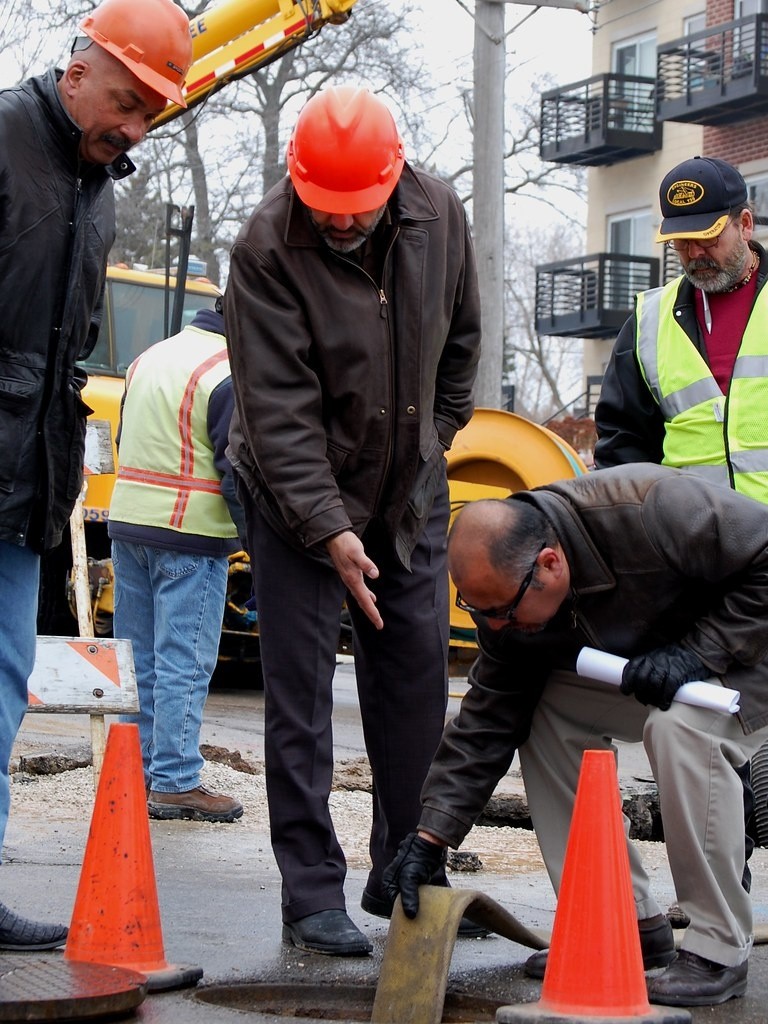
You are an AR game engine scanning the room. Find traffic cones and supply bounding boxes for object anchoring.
[496,750,692,1024]
[60,723,204,995]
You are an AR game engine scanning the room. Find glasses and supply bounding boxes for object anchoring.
[667,216,738,251]
[455,541,548,624]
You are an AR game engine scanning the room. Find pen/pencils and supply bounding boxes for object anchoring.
[701,290,712,335]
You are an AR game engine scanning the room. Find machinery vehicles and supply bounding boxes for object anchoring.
[46,0,588,689]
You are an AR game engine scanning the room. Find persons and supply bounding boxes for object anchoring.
[0,0,197,950]
[382,462,768,1003]
[594,156,768,923]
[108,297,245,822]
[220,87,481,953]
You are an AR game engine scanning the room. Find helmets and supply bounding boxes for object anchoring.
[286,86,404,215]
[78,0,193,111]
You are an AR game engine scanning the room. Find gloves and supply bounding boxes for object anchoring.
[383,833,448,922]
[615,636,715,712]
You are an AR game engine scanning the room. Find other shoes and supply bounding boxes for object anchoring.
[0,903,70,950]
[148,785,244,824]
[664,898,691,928]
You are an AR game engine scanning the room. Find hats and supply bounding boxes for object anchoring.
[656,155,748,243]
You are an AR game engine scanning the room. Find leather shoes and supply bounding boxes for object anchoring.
[282,908,374,955]
[360,867,494,938]
[521,918,677,980]
[649,949,749,1007]
